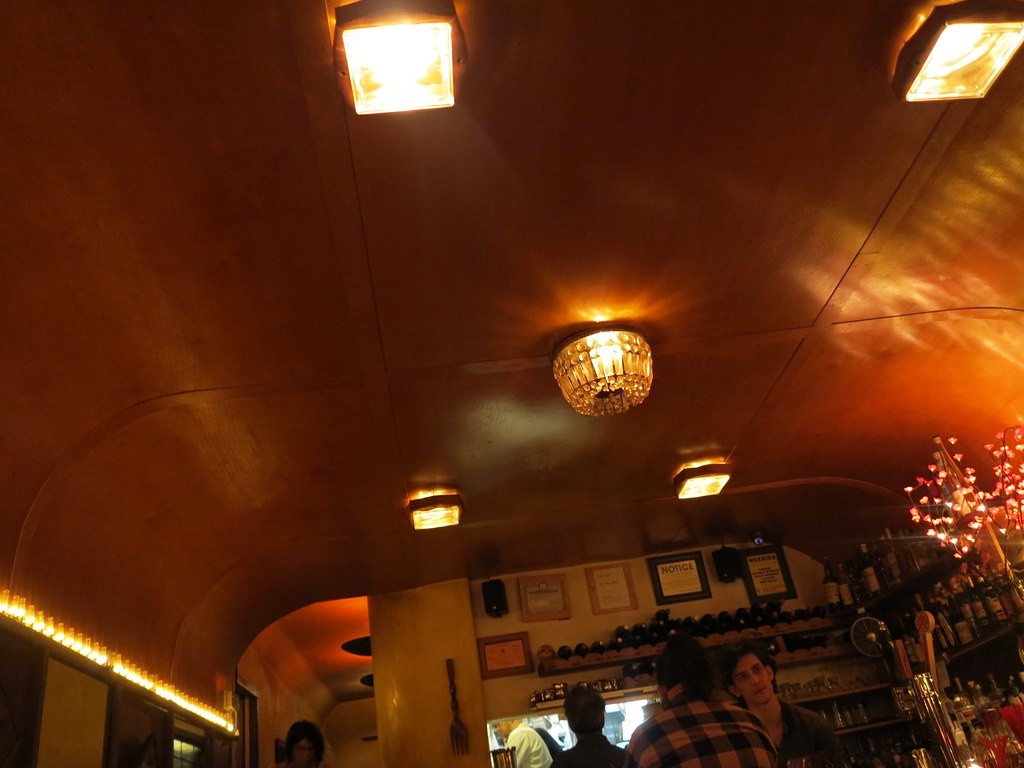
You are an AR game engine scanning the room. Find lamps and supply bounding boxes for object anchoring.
[405,486,465,530]
[889,0,1024,105]
[550,323,656,419]
[324,0,469,115]
[673,459,732,500]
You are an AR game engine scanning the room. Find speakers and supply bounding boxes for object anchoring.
[712,546,743,583]
[481,579,509,618]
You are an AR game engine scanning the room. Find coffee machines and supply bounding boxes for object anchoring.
[894,575,1017,664]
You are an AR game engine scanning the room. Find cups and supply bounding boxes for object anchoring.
[780,677,833,701]
[544,676,623,701]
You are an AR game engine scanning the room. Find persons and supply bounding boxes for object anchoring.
[550,686,627,768]
[271,720,325,768]
[720,638,848,768]
[493,718,564,768]
[624,631,780,768]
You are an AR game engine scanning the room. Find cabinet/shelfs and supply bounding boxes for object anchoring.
[516,530,1024,768]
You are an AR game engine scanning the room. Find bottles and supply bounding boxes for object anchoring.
[819,701,869,730]
[557,640,607,660]
[822,544,883,608]
[880,525,924,579]
[841,728,945,768]
[607,599,826,675]
[953,672,1024,724]
